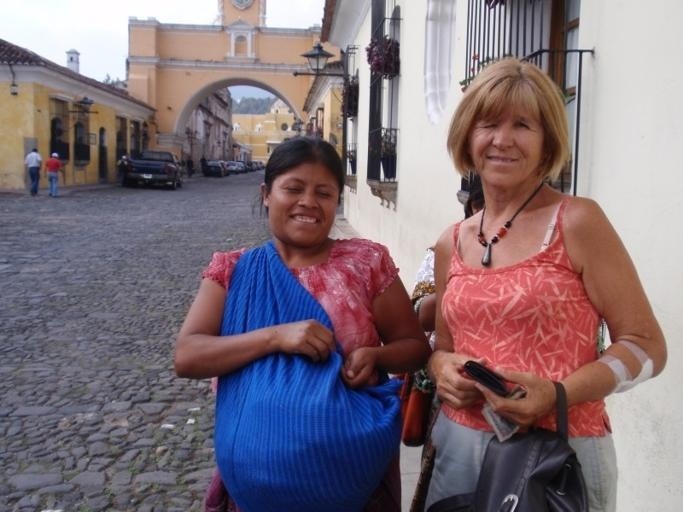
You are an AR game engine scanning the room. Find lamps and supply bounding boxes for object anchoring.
[69,96,98,114]
[294,42,345,76]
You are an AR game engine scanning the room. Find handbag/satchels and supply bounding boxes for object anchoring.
[472,379,588,512]
[392,294,435,446]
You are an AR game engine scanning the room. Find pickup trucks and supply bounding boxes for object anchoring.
[126,150,183,190]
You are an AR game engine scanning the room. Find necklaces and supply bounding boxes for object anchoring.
[477,179,544,267]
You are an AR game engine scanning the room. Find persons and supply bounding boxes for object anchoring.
[44,152,61,197]
[410,171,486,511]
[424,58,667,512]
[173,138,431,512]
[24,148,42,197]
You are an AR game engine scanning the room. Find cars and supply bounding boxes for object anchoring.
[204,160,264,178]
